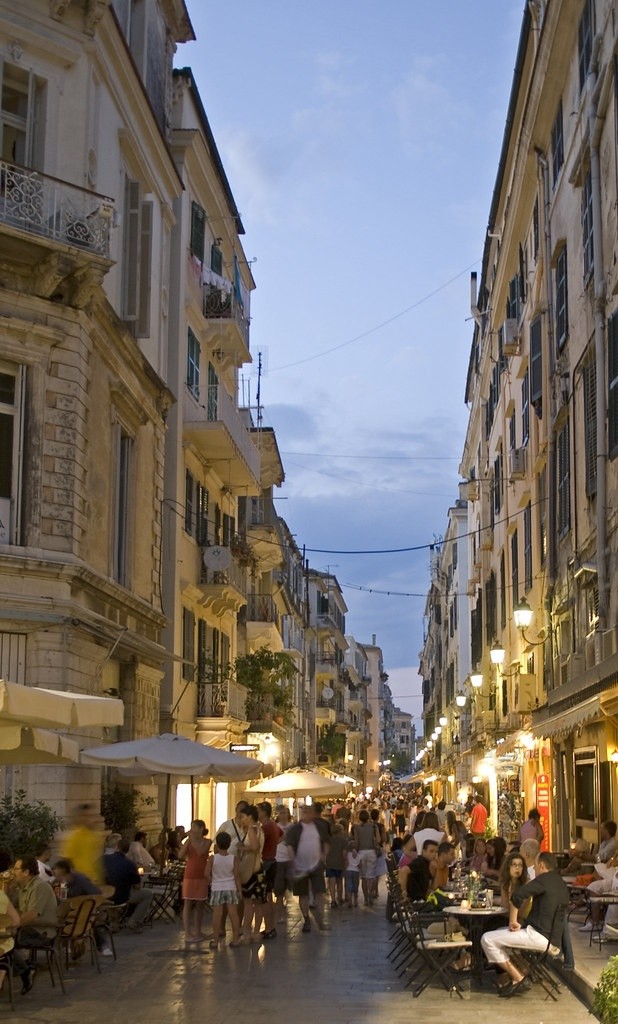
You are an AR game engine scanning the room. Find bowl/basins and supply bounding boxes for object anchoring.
[472,903,486,908]
[580,863,597,874]
[443,881,455,891]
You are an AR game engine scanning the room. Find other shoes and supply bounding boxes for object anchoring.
[184,891,380,953]
[448,961,476,970]
[101,948,112,956]
[579,922,603,931]
[592,936,610,943]
[499,977,533,997]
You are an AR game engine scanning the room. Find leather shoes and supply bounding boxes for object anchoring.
[20,967,35,995]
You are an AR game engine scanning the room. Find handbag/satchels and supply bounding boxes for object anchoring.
[373,824,383,858]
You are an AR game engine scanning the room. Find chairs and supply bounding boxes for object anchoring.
[0,884,126,1012]
[388,843,618,1001]
[129,857,213,928]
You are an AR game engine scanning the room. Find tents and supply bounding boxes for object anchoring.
[0,678,125,765]
[530,686,618,757]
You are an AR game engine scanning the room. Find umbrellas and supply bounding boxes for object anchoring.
[80,734,274,879]
[241,765,347,823]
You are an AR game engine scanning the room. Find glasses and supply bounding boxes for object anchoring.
[13,866,26,871]
[511,863,525,868]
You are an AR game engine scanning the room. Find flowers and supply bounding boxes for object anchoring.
[464,867,486,888]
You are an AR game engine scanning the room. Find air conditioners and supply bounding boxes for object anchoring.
[467,481,476,501]
[473,564,482,582]
[479,528,493,549]
[502,316,518,354]
[508,448,524,479]
[466,578,475,596]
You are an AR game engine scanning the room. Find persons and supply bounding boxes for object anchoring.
[344,784,488,913]
[399,808,618,996]
[99,819,212,943]
[55,803,113,956]
[204,797,387,949]
[0,843,104,997]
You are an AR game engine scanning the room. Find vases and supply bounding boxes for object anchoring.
[466,885,480,907]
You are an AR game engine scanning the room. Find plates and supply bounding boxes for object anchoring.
[469,908,498,912]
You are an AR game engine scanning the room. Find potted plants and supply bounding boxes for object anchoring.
[203,641,301,724]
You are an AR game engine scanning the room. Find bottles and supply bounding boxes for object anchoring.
[176,832,191,845]
[60,883,67,899]
[54,883,60,899]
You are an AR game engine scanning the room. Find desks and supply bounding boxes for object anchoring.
[134,867,159,877]
[439,904,507,984]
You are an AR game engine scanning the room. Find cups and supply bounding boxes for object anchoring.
[49,876,56,883]
[0,868,14,882]
[487,890,493,908]
[479,894,486,902]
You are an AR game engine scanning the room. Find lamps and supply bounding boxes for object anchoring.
[411,712,458,764]
[213,347,224,359]
[359,758,364,765]
[470,667,499,697]
[348,752,353,761]
[490,640,525,677]
[456,690,475,706]
[612,750,618,761]
[265,733,270,743]
[212,237,223,248]
[513,595,552,645]
